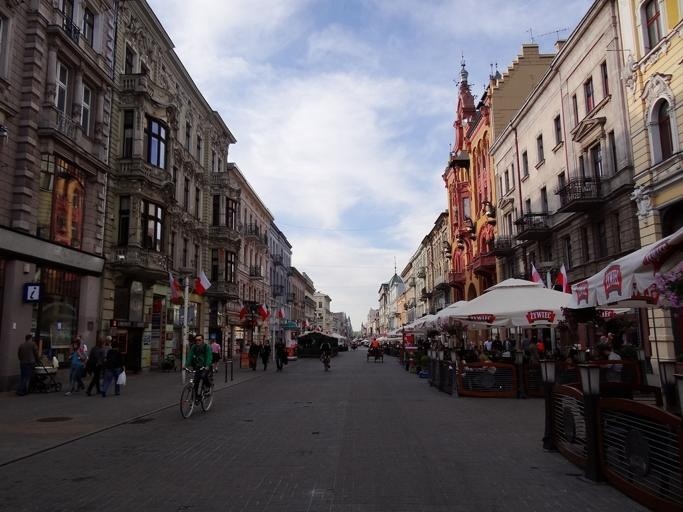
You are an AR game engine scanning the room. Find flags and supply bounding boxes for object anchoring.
[556,264,570,293]
[531,266,546,289]
[168,269,286,320]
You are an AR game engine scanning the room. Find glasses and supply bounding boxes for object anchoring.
[195,339,203,342]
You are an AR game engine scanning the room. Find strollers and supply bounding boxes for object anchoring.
[32,355,65,393]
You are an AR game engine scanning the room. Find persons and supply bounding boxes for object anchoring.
[473,331,632,380]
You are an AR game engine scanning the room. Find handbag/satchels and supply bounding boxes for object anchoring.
[116,370,126,384]
[79,365,87,377]
[86,359,94,376]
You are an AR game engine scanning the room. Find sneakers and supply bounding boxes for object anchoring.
[114,390,121,395]
[250,365,285,372]
[64,390,73,397]
[213,367,218,373]
[203,386,211,397]
[84,389,91,397]
[101,389,107,398]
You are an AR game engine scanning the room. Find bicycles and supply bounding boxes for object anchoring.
[175,365,216,418]
[320,349,330,372]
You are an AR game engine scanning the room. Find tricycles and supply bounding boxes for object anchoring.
[365,348,384,364]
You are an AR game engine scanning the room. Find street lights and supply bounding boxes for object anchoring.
[177,265,194,383]
[270,305,277,362]
